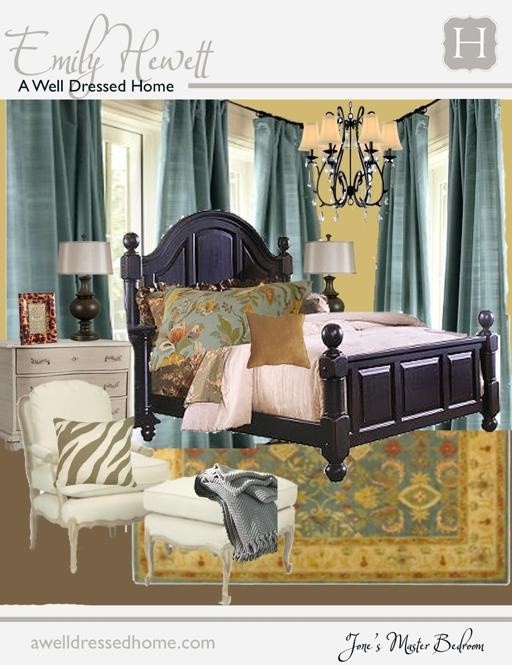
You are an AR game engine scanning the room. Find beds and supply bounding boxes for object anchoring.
[120,210,501,482]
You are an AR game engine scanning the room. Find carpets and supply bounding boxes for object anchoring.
[128,429,508,586]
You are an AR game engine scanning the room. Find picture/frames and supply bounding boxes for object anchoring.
[18,292,58,346]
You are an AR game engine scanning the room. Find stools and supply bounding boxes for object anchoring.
[145,472,298,606]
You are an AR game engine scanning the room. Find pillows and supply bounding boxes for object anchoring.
[245,312,311,371]
[148,279,313,371]
[55,416,139,493]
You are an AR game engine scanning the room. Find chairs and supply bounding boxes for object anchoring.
[15,380,172,574]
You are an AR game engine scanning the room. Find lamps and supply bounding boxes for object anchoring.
[56,233,113,342]
[298,100,404,223]
[304,233,356,312]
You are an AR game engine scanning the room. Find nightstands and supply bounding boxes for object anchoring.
[0,339,132,452]
